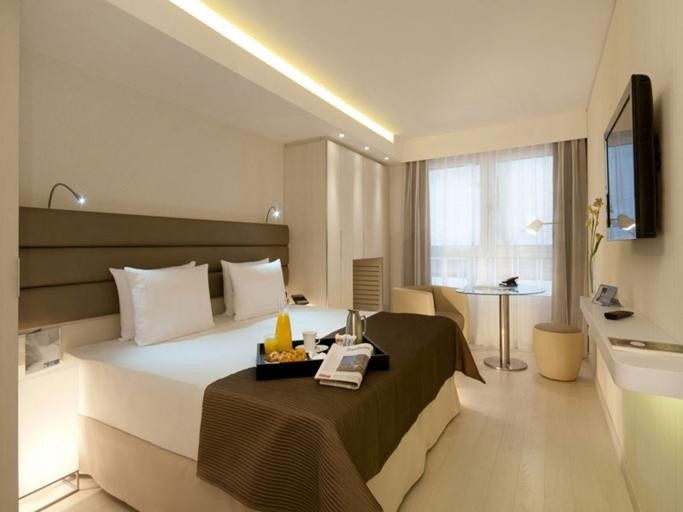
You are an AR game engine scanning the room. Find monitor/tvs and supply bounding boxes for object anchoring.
[603,74,661,242]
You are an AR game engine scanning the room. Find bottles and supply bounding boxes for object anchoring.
[275,311,292,352]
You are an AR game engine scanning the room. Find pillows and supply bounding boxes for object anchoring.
[105,255,290,344]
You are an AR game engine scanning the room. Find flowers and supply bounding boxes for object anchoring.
[584,197,605,295]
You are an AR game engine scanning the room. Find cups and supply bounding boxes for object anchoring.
[264,337,278,354]
[303,331,320,350]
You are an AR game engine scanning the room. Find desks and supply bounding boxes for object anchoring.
[456,283,546,371]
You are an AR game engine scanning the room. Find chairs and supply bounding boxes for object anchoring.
[389,285,467,340]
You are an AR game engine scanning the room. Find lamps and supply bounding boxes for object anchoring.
[611,215,635,230]
[523,218,562,234]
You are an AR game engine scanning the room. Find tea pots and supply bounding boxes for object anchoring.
[346,309,367,344]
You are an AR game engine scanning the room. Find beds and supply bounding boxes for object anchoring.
[16,206,468,511]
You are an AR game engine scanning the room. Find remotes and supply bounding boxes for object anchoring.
[604,311,634,320]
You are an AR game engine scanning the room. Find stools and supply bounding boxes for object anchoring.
[533,322,585,383]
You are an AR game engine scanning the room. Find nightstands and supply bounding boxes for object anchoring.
[17,354,90,509]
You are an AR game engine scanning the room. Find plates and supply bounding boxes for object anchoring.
[296,344,329,353]
